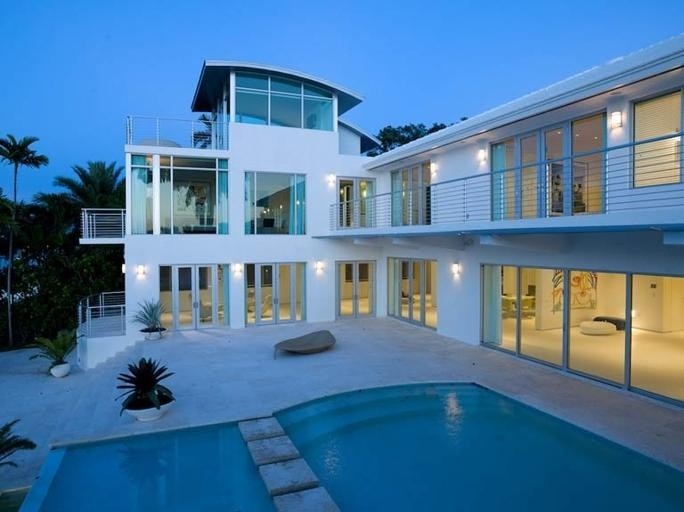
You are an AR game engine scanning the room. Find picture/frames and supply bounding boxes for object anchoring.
[546,158,590,217]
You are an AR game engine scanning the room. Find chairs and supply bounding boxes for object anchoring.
[189,289,272,323]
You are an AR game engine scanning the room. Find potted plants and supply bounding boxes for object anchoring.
[127,298,169,340]
[26,329,85,378]
[115,357,176,422]
